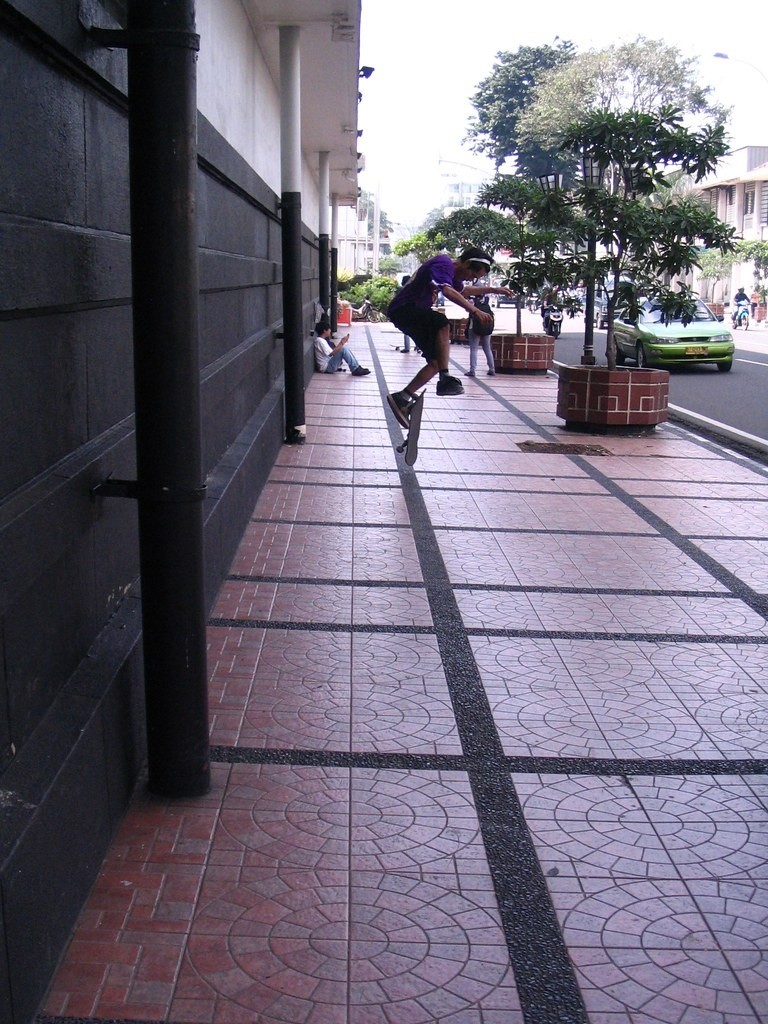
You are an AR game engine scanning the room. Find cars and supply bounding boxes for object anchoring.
[587,299,641,329]
[558,294,584,303]
[613,295,736,373]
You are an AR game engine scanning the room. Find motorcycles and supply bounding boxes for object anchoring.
[337,293,381,323]
[527,293,541,314]
[539,304,563,339]
[730,301,757,330]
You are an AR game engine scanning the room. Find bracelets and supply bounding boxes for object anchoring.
[341,341,343,346]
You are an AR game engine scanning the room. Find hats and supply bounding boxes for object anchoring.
[474,283,484,287]
[402,276,410,283]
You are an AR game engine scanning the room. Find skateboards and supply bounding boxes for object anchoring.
[396,388,427,466]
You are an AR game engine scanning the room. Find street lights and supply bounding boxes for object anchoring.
[537,152,647,366]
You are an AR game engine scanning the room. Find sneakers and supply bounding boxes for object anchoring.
[387,393,412,429]
[437,376,465,395]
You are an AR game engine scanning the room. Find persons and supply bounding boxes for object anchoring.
[733,287,750,320]
[400,276,421,354]
[314,321,371,376]
[541,283,559,331]
[386,246,517,429]
[464,282,496,377]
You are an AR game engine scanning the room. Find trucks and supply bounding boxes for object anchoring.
[488,274,525,309]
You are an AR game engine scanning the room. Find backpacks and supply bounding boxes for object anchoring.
[473,296,494,335]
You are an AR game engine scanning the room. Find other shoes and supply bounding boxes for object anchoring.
[464,372,475,376]
[545,327,548,331]
[400,349,408,353]
[352,365,371,376]
[414,347,421,353]
[487,371,495,376]
